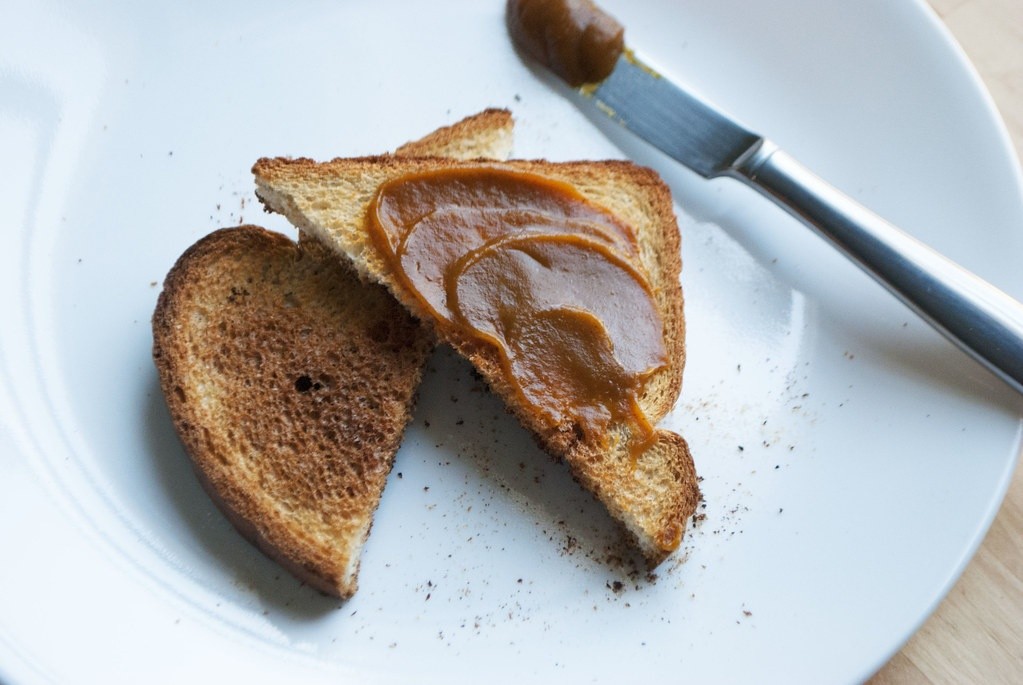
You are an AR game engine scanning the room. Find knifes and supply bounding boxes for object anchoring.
[512,0,1023,397]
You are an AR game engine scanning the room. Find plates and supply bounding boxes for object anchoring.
[0,0,1023,685]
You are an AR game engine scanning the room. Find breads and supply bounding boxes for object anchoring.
[153,105,515,602]
[252,153,698,563]
